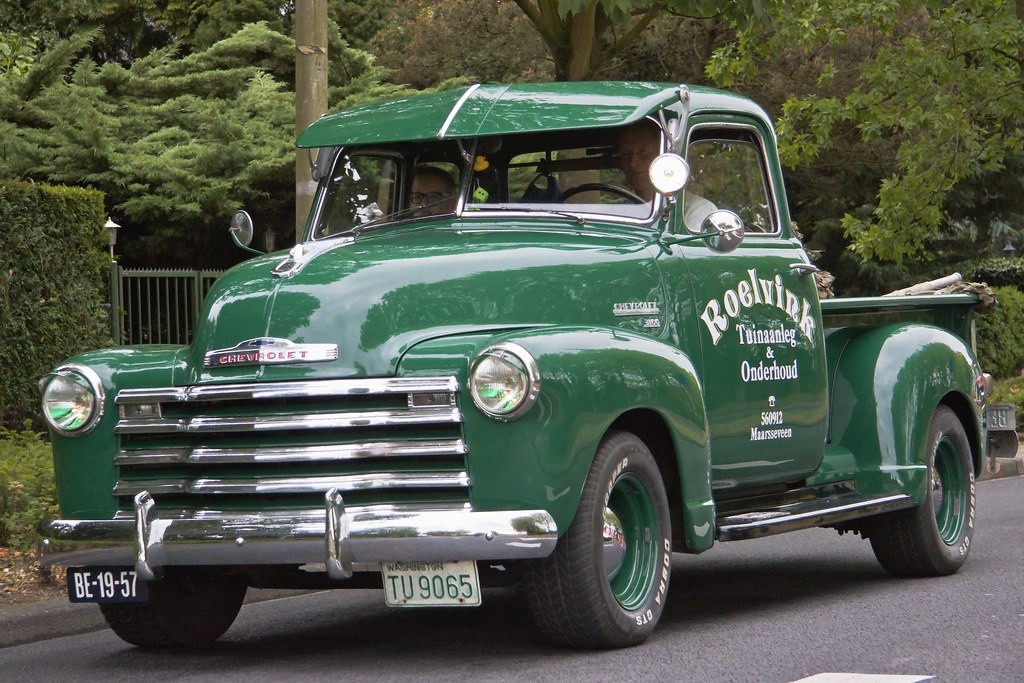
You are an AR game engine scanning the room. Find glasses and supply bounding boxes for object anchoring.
[408,189,452,205]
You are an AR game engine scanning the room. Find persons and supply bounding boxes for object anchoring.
[409,165,457,218]
[617,124,719,231]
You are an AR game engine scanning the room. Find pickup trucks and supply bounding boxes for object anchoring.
[34,78,994,652]
